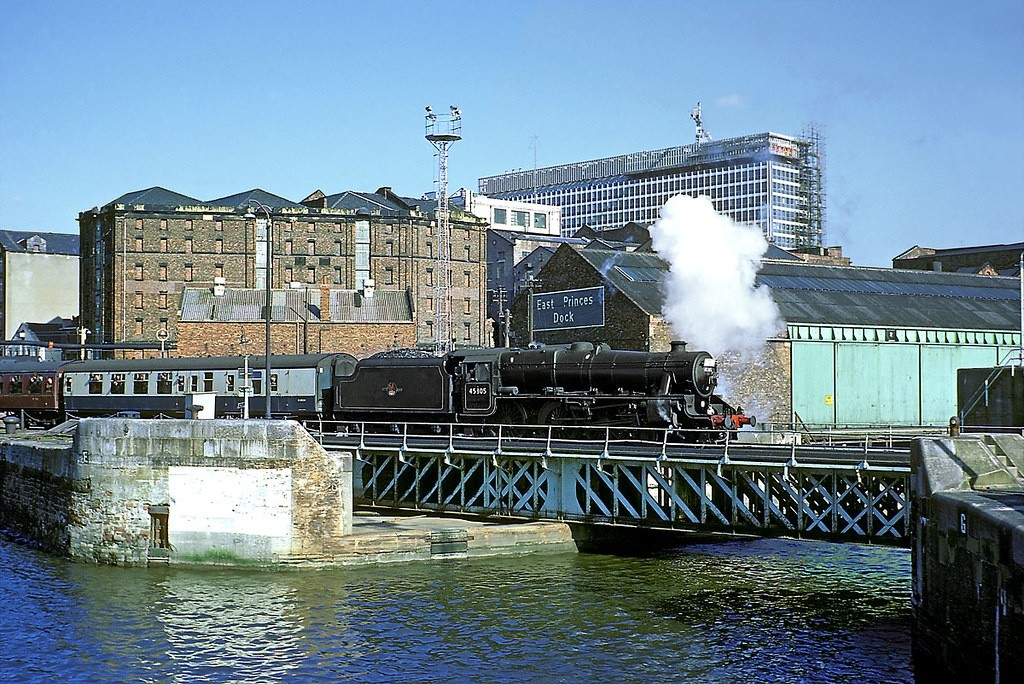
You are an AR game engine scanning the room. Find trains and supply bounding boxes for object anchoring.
[0,338,757,444]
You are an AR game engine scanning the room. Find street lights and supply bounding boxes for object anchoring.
[424,104,463,356]
[242,197,290,418]
